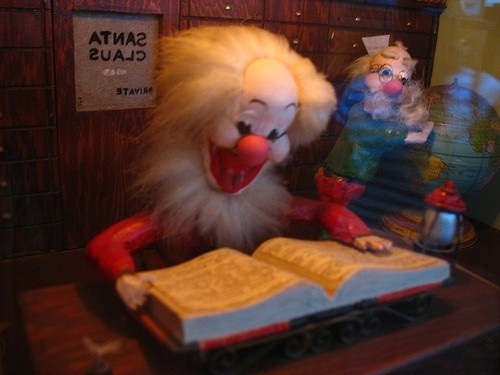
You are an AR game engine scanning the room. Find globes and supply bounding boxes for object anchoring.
[377,76,500,250]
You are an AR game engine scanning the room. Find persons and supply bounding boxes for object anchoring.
[313,45,436,242]
[81,24,393,314]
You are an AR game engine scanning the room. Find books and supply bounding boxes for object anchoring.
[126,239,450,350]
